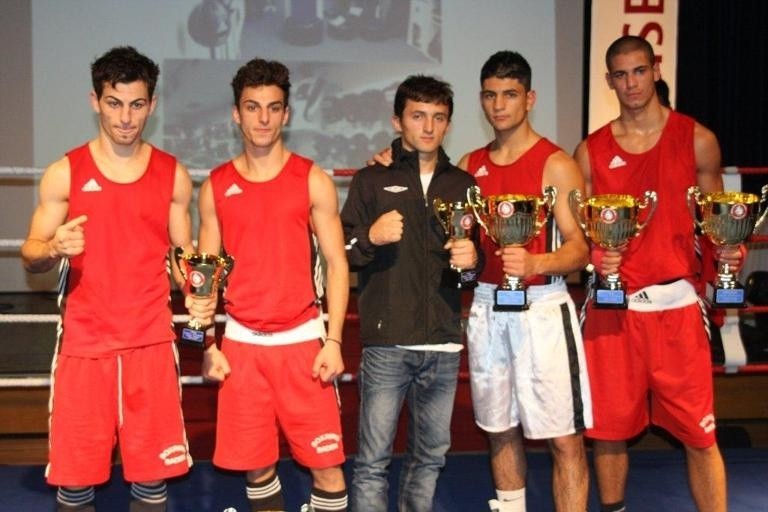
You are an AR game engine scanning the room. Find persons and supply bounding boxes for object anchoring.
[363,49,590,512]
[339,73,486,511]
[21,43,219,512]
[572,35,748,511]
[197,57,349,512]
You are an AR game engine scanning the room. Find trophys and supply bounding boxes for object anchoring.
[686,184,768,303]
[173,245,235,349]
[568,187,659,309]
[466,184,558,312]
[431,197,481,291]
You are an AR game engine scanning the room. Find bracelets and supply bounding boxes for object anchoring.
[326,337,342,347]
